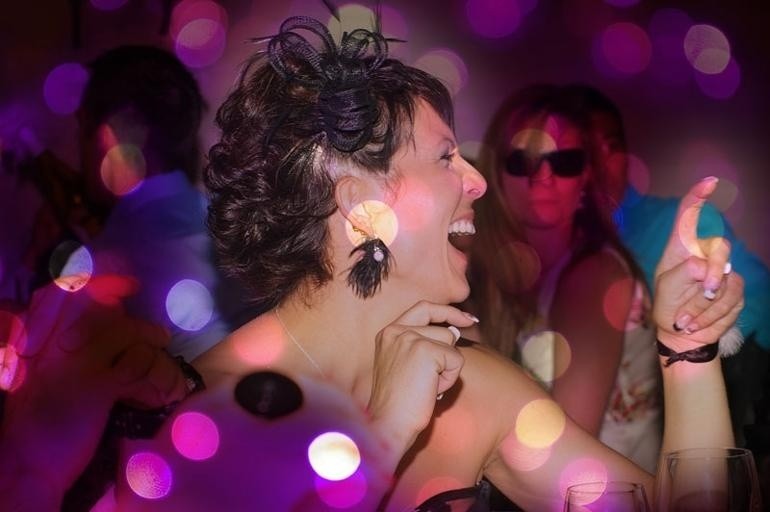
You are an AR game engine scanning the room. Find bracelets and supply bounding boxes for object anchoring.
[656,337,720,368]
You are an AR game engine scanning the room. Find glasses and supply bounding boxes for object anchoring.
[501,146,585,177]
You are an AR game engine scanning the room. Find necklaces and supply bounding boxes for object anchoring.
[275,302,327,383]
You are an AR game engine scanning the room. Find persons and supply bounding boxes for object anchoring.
[0,45,232,512]
[190,16,746,512]
[411,79,770,512]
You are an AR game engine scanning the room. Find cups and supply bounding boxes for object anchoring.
[561,481,651,512]
[655,446,761,511]
